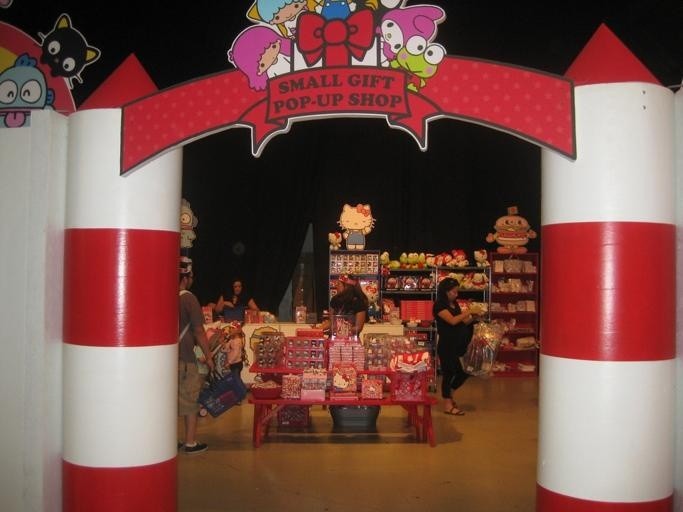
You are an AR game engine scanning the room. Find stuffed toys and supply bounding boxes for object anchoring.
[328,232,343,250]
[379,245,491,291]
[362,282,380,323]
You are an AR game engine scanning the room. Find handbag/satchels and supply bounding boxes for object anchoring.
[198,373,247,418]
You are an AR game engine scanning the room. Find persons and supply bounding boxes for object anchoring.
[433,277,473,416]
[178,255,215,455]
[222,320,248,405]
[215,279,258,321]
[330,271,369,334]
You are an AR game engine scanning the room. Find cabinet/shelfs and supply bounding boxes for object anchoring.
[328,248,382,325]
[380,265,437,382]
[432,264,492,378]
[247,357,440,449]
[488,253,539,378]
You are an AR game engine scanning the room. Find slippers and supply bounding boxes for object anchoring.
[444,402,465,416]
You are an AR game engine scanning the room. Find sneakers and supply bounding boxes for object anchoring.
[184,441,208,454]
